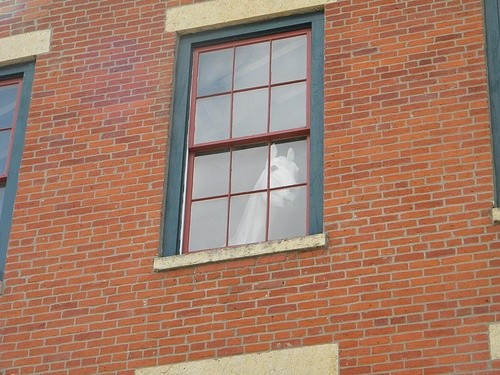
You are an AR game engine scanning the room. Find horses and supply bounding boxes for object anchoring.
[224,143,299,247]
